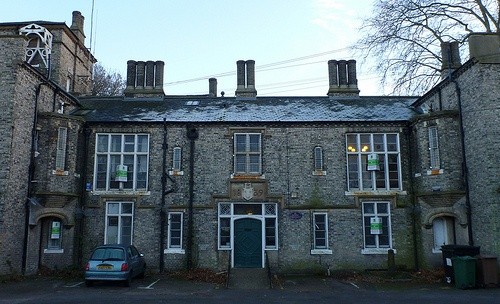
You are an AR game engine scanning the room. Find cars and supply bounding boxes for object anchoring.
[85,244,146,287]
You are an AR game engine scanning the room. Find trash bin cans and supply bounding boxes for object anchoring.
[453,254,485,291]
[439,244,480,289]
